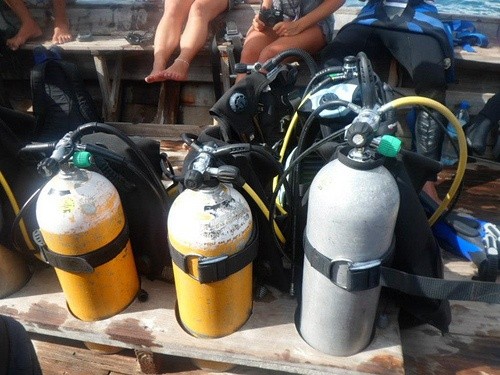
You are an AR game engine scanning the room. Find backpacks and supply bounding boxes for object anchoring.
[21,44,100,142]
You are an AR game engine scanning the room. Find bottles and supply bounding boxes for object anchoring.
[440,101,470,166]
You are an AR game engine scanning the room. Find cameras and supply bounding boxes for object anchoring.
[259,9,284,25]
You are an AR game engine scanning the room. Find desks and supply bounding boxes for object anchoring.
[0,264,500,375]
[382,41,500,89]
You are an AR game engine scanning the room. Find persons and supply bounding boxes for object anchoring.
[3,0,74,51]
[144,0,229,84]
[465,87,500,156]
[312,0,453,213]
[235,0,346,87]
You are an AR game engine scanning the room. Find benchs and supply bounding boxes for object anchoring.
[9,27,232,141]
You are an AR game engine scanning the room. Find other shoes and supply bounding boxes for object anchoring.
[463,111,495,157]
